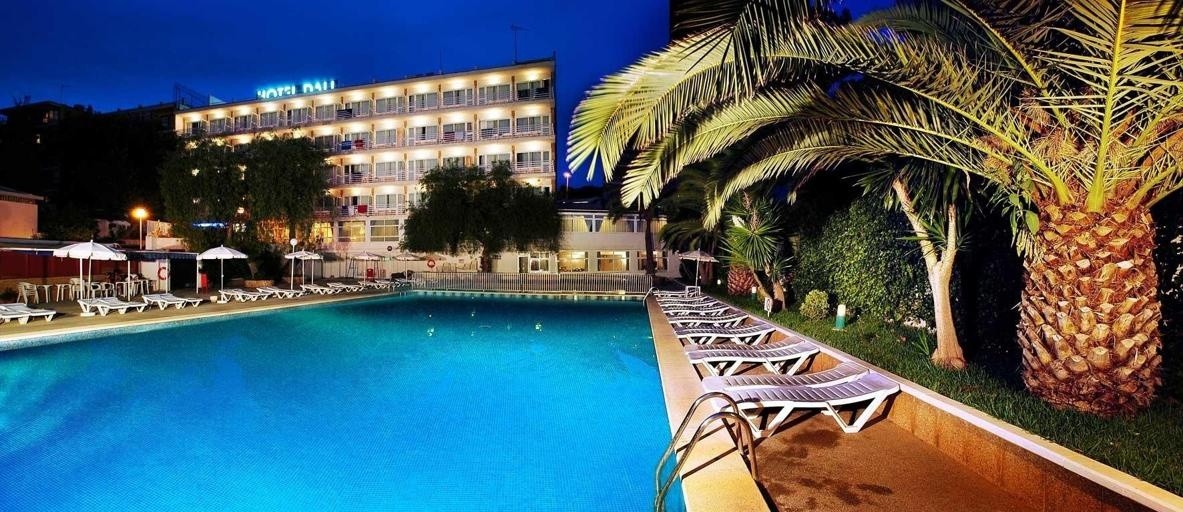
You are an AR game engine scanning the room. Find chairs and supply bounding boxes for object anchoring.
[216,278,413,303]
[0,273,202,325]
[653,290,900,438]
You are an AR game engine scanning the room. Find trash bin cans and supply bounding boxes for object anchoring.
[367,269,373,277]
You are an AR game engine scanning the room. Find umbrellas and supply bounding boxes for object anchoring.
[353,250,385,286]
[392,250,426,283]
[285,248,324,295]
[195,244,248,304]
[52,235,127,315]
[676,248,721,289]
[298,252,325,285]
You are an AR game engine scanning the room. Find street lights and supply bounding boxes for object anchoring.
[288,237,298,290]
[129,203,149,250]
